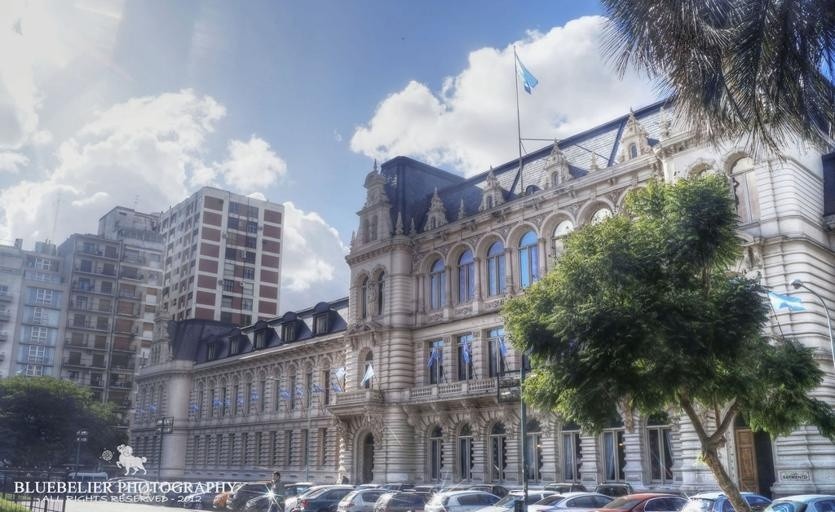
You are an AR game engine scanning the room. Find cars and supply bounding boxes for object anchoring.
[0,470,834,511]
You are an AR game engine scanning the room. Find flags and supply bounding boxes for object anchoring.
[132,366,375,413]
[426,337,509,369]
[769,290,807,315]
[516,53,539,95]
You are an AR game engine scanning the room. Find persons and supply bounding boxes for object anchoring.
[267,472,284,512]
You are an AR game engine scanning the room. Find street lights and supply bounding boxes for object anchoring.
[790,279,834,366]
[153,417,174,478]
[73,430,88,471]
